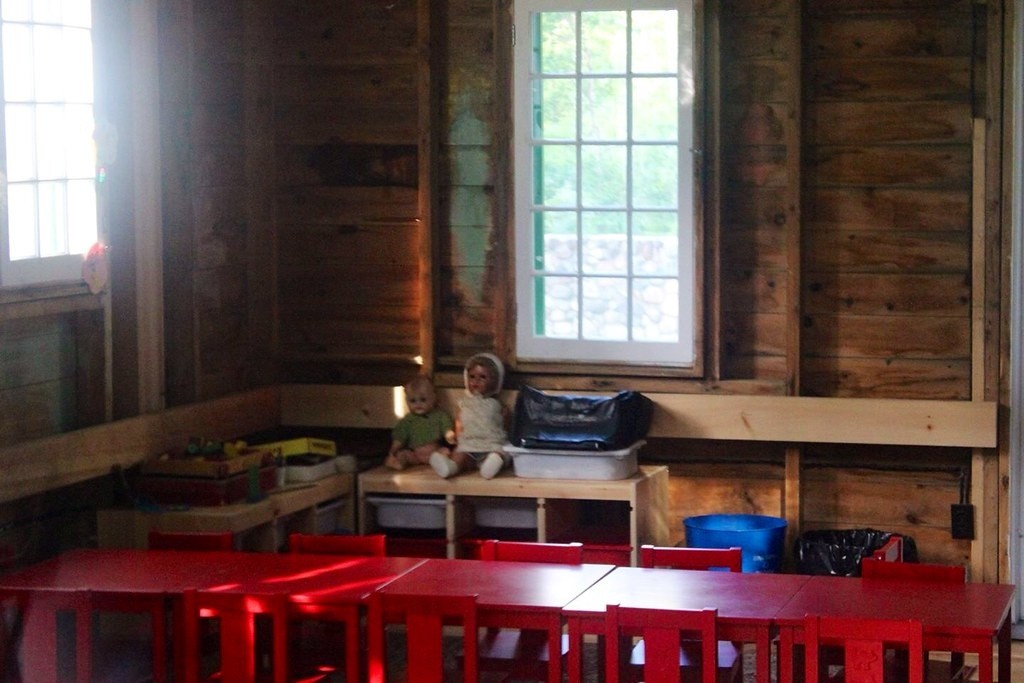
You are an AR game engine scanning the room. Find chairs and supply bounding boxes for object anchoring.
[0,525,979,683]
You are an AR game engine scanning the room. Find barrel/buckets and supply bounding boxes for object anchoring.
[683,514,788,574]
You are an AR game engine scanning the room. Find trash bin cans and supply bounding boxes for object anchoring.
[683,513,788,573]
[799,527,912,577]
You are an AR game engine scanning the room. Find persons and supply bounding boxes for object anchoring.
[429,353,511,480]
[385,375,455,471]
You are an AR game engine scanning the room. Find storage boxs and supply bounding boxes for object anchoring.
[131,438,337,506]
[364,495,474,528]
[317,500,345,534]
[502,441,647,480]
[473,499,589,527]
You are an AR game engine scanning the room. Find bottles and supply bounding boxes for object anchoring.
[188,443,202,455]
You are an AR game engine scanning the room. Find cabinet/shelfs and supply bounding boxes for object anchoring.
[357,466,670,645]
[97,473,356,636]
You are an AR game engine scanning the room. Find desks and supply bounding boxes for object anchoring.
[169,552,428,683]
[364,559,617,682]
[0,547,247,683]
[776,576,1016,683]
[562,567,812,682]
